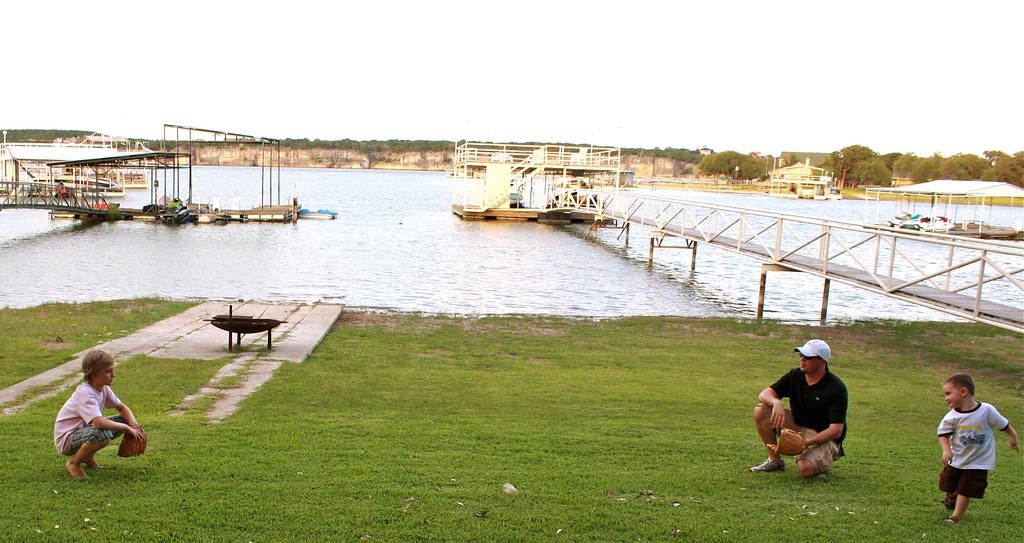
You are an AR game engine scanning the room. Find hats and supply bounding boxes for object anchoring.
[793,339,831,363]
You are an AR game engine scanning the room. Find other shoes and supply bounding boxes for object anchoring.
[66,461,88,481]
[84,457,99,470]
[946,514,959,524]
[944,494,956,510]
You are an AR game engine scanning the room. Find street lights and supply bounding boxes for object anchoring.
[2,129,8,148]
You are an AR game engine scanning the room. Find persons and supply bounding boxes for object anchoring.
[54,349,143,479]
[937,373,1019,524]
[750,339,848,478]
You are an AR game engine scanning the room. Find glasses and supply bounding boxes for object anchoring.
[799,352,813,360]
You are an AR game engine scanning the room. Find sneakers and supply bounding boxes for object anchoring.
[750,458,785,472]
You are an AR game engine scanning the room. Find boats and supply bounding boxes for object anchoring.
[158,202,191,226]
[96,203,121,209]
[546,177,605,223]
[297,209,339,220]
[798,184,843,201]
[889,212,955,233]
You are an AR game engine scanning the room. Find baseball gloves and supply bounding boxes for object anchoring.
[766,428,806,456]
[117,424,147,457]
